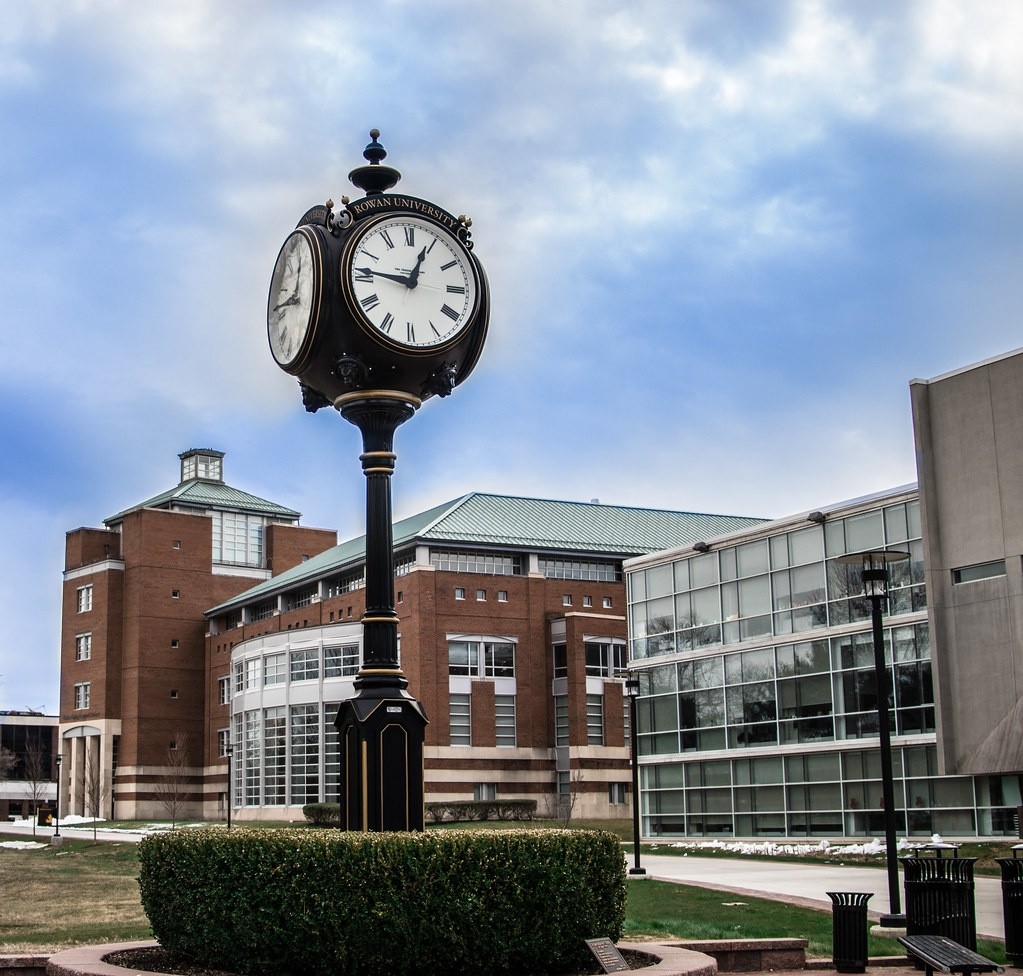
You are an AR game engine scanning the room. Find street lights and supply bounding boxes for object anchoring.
[51,754,67,836]
[609,668,655,876]
[219,743,241,831]
[834,549,913,929]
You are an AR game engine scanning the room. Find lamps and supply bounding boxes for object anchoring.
[691,541,709,554]
[808,512,827,523]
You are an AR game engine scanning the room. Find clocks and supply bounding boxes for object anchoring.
[267,229,313,365]
[349,215,476,348]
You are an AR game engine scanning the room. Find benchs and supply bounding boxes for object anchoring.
[896,935,1006,976]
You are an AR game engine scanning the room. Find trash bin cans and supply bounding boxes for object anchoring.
[826,891,874,974]
[994,843,1023,969]
[898,844,979,971]
